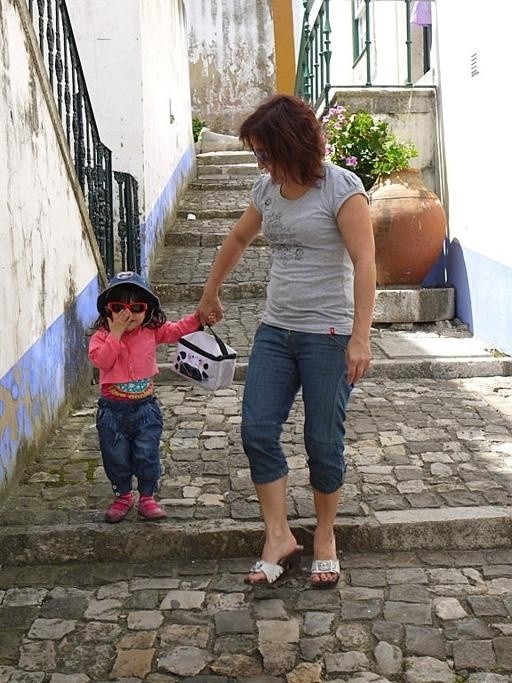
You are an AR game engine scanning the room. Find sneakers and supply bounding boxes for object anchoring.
[105,491,134,522]
[138,494,168,522]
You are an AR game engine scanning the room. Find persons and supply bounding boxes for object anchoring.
[193,94,378,587]
[88,266,217,523]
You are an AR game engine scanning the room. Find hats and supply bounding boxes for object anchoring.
[97,271,162,329]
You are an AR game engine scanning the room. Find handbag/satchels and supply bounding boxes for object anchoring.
[171,321,238,391]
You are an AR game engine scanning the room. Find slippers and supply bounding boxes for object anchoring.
[311,558,340,587]
[244,545,304,584]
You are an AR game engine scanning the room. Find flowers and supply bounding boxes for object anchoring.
[323,105,406,176]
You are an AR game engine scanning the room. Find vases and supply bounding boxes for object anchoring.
[356,170,450,288]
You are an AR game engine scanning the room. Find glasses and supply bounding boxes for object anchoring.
[253,149,273,161]
[107,302,148,313]
